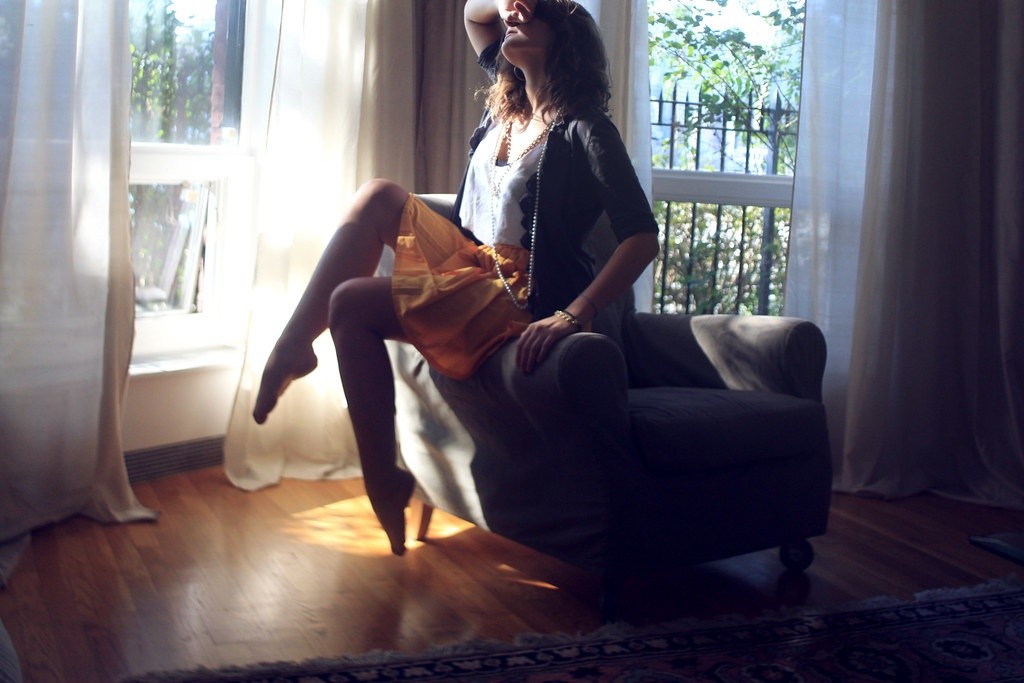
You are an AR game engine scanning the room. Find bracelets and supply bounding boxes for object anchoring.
[555,310,582,332]
[578,294,599,318]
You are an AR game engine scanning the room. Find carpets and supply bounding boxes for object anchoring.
[115,582,1024,683]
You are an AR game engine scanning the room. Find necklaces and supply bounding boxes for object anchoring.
[490,105,564,309]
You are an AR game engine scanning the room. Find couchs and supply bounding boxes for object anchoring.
[376,192,833,611]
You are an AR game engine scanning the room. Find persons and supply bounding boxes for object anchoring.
[253,0,660,555]
